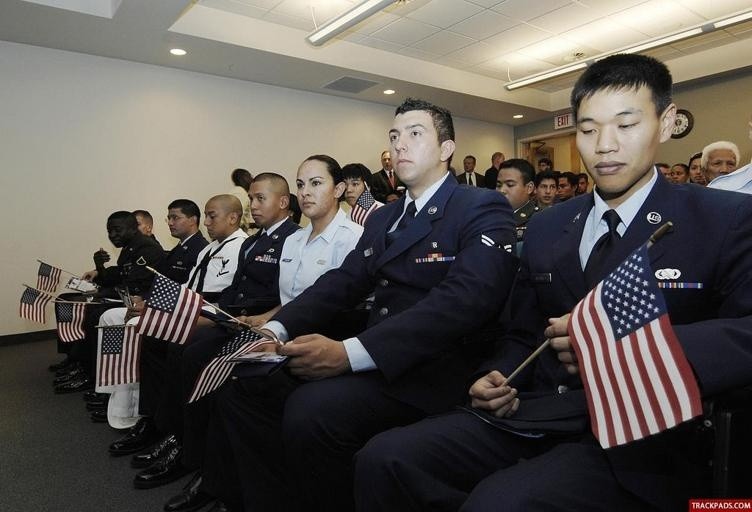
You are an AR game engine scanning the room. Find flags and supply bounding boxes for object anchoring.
[19,261,267,403]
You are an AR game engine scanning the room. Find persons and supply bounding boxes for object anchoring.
[49,52,752,512]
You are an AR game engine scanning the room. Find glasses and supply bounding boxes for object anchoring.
[164,216,192,223]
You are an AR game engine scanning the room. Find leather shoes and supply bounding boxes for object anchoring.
[55,372,92,394]
[208,500,234,511]
[91,408,108,422]
[86,401,108,410]
[133,447,191,490]
[108,420,161,458]
[164,473,217,512]
[130,433,182,468]
[83,391,108,401]
[50,359,83,384]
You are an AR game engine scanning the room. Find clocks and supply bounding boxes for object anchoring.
[670,109,694,139]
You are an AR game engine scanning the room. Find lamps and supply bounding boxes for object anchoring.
[502,7,752,92]
[304,0,410,49]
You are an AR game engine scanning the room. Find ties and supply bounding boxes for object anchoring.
[389,171,392,180]
[584,209,623,275]
[468,173,473,186]
[247,233,266,259]
[396,200,417,232]
